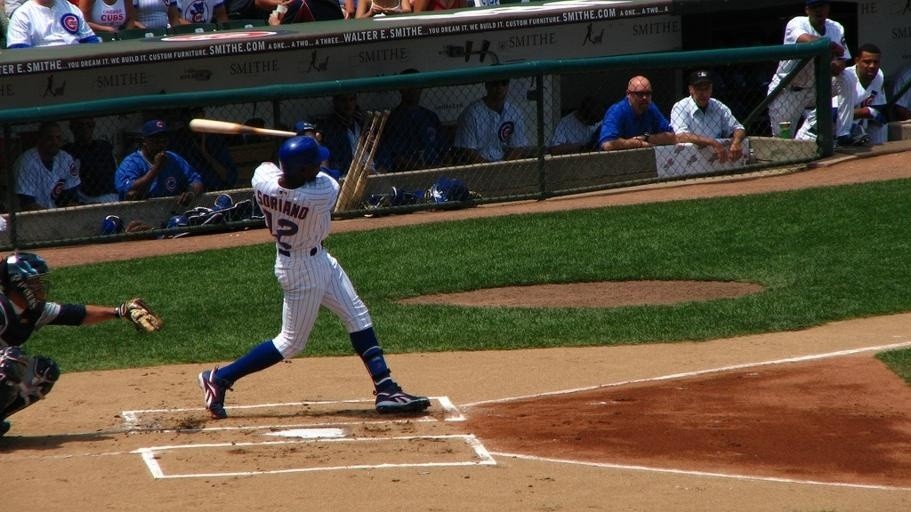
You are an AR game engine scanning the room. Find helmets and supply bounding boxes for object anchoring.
[278,136,329,172]
[0,251,51,315]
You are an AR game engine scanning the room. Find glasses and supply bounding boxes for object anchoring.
[632,90,651,97]
[149,138,167,144]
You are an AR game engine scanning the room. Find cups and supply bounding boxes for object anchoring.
[780,122,792,137]
[273,5,289,24]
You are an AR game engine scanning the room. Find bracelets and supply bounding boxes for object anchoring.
[112,305,119,319]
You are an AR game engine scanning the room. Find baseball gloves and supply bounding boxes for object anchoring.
[116,298,164,334]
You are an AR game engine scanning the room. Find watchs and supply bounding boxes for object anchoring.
[643,130,649,141]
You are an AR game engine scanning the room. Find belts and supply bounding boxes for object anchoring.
[278,240,325,257]
[791,85,804,92]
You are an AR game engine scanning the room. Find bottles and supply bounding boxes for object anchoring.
[854,90,879,109]
[749,149,757,163]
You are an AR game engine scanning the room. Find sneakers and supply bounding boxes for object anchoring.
[373,386,431,414]
[198,365,228,419]
[837,134,871,146]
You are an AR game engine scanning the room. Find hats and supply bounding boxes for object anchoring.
[142,120,165,136]
[294,121,316,135]
[689,71,714,86]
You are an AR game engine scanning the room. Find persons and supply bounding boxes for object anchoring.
[197,133,433,420]
[2,2,529,215]
[796,44,911,147]
[766,1,872,149]
[547,91,608,158]
[670,64,750,164]
[600,73,676,151]
[0,242,161,444]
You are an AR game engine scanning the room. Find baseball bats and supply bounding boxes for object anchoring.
[189,119,298,136]
[333,110,390,219]
